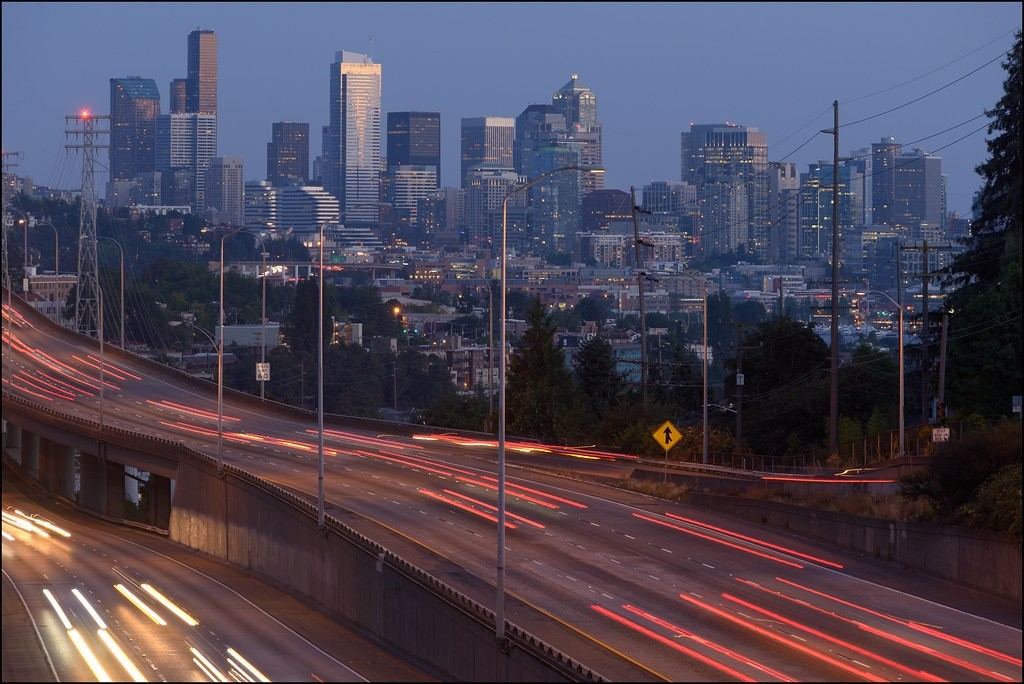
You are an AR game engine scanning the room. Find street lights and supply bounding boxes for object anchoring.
[319,202,392,528]
[80,234,124,351]
[168,320,219,356]
[452,266,494,435]
[217,220,275,474]
[664,267,708,463]
[856,288,904,459]
[19,218,58,323]
[496,164,607,643]
[42,269,103,430]
[200,227,265,402]
